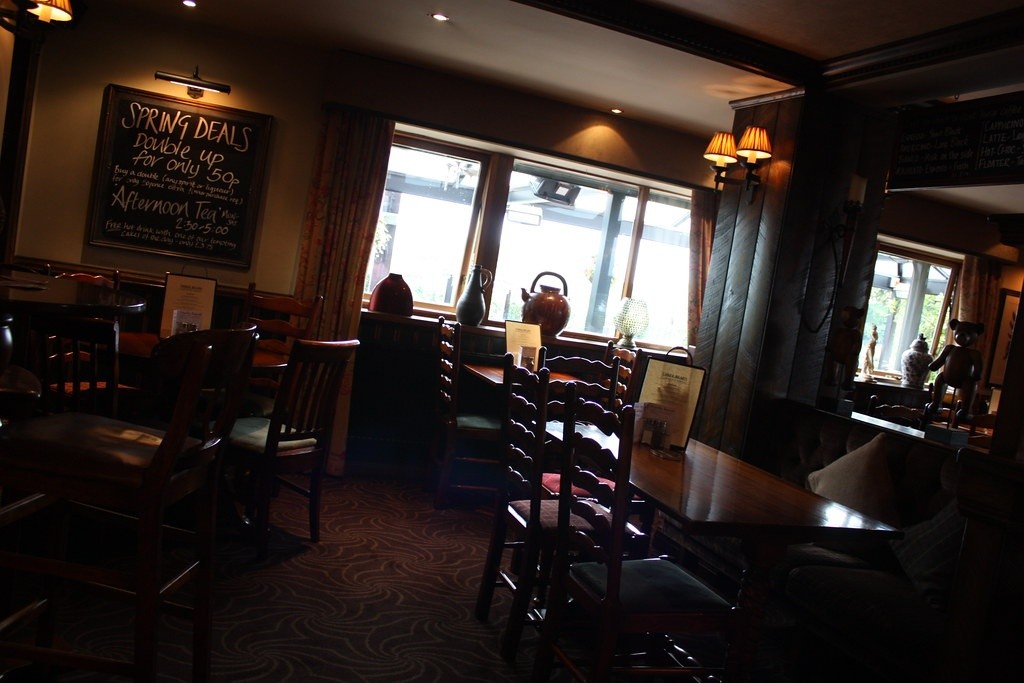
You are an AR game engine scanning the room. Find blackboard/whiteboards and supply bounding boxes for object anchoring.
[84,81,278,272]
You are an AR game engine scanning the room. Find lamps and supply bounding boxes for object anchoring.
[27,0,73,24]
[704,132,737,176]
[155,68,230,99]
[740,125,771,171]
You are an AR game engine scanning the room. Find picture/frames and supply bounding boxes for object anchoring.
[987,288,1022,387]
[88,83,278,270]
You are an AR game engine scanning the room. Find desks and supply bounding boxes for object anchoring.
[542,415,905,683]
[460,361,590,393]
[850,373,933,408]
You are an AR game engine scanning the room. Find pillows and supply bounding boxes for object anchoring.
[893,497,1022,618]
[805,433,900,524]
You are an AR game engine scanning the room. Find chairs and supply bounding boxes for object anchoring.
[537,347,618,473]
[534,381,731,683]
[2,263,357,682]
[436,354,510,509]
[472,361,645,662]
[866,394,995,450]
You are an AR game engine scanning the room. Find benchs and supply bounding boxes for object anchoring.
[654,402,1022,682]
